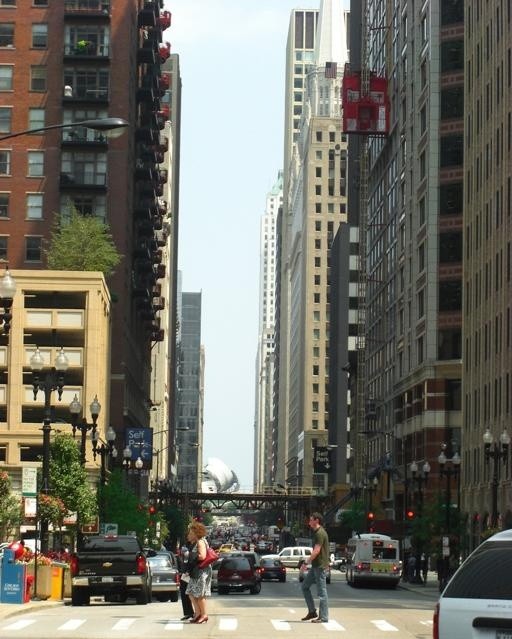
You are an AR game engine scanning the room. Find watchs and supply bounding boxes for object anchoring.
[302,560,308,565]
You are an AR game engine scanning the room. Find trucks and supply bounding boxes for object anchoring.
[344,529,404,589]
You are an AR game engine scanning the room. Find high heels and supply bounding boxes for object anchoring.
[190,615,209,624]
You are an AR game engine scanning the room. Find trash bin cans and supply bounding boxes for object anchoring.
[28,562,70,601]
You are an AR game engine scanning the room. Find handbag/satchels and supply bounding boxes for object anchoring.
[196,538,219,569]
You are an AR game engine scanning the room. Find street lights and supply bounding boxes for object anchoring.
[28,343,71,606]
[483,424,512,531]
[276,482,287,525]
[351,463,432,588]
[356,427,407,522]
[434,450,461,536]
[322,442,367,458]
[68,390,213,548]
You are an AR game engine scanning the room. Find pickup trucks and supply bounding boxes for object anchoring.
[66,530,153,607]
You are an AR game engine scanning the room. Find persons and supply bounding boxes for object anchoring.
[180,533,197,621]
[408,555,463,588]
[185,521,212,623]
[300,512,329,623]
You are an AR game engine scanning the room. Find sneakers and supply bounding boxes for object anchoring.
[180,614,193,621]
[301,609,319,621]
[311,618,325,623]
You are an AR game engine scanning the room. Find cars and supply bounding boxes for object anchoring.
[0,536,42,560]
[147,520,347,604]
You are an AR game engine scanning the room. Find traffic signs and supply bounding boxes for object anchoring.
[312,446,338,474]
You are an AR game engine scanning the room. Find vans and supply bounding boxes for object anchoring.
[429,526,512,639]
